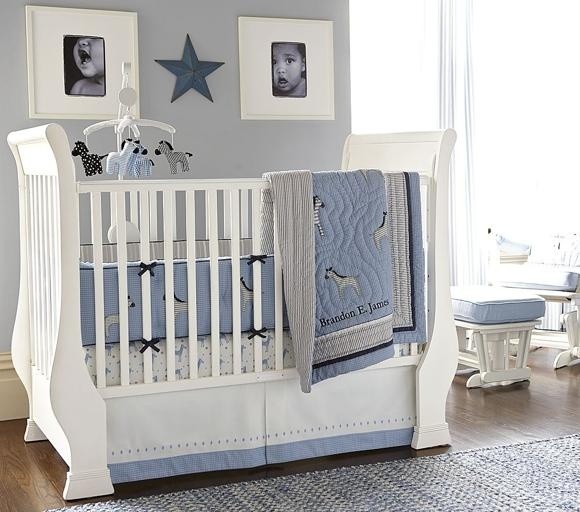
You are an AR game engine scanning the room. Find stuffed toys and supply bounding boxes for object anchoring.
[71,137,194,178]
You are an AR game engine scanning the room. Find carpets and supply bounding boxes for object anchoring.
[44,427,580,511]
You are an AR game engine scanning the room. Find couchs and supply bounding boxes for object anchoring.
[484,218,580,371]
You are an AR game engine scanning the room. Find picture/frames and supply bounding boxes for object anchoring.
[24,3,140,122]
[237,16,335,121]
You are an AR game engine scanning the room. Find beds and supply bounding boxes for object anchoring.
[7,122,460,503]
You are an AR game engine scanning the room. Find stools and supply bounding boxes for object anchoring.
[451,284,546,391]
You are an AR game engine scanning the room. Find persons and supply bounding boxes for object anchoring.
[69,35,106,96]
[271,42,307,98]
